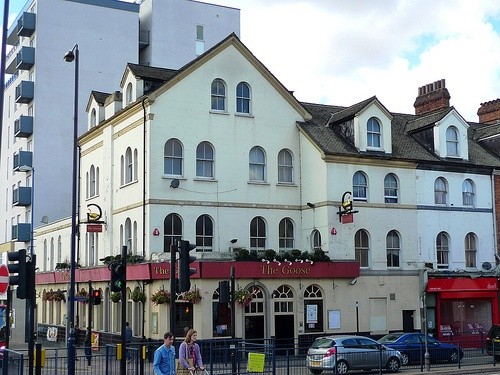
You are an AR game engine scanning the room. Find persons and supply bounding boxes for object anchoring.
[73,324,81,361]
[126,322,133,364]
[0,325,6,340]
[84,326,90,359]
[179,329,204,373]
[153,332,176,375]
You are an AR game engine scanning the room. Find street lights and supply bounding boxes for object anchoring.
[63,44,80,375]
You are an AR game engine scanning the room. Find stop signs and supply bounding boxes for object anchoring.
[0,264,10,295]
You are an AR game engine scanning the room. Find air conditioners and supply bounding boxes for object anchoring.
[481,261,497,272]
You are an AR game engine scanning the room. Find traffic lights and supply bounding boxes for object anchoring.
[110,262,126,281]
[8,249,26,298]
[177,240,196,292]
[94,290,101,304]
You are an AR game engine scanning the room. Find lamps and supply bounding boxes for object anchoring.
[284,259,292,265]
[262,259,270,264]
[295,259,303,263]
[307,203,314,208]
[230,239,237,243]
[305,259,314,264]
[273,259,281,266]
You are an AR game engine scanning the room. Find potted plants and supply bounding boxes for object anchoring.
[151,290,169,306]
[131,287,146,303]
[110,292,121,303]
[185,290,203,304]
[234,288,253,305]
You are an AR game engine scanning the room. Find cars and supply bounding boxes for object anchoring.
[377,332,464,365]
[306,335,404,374]
